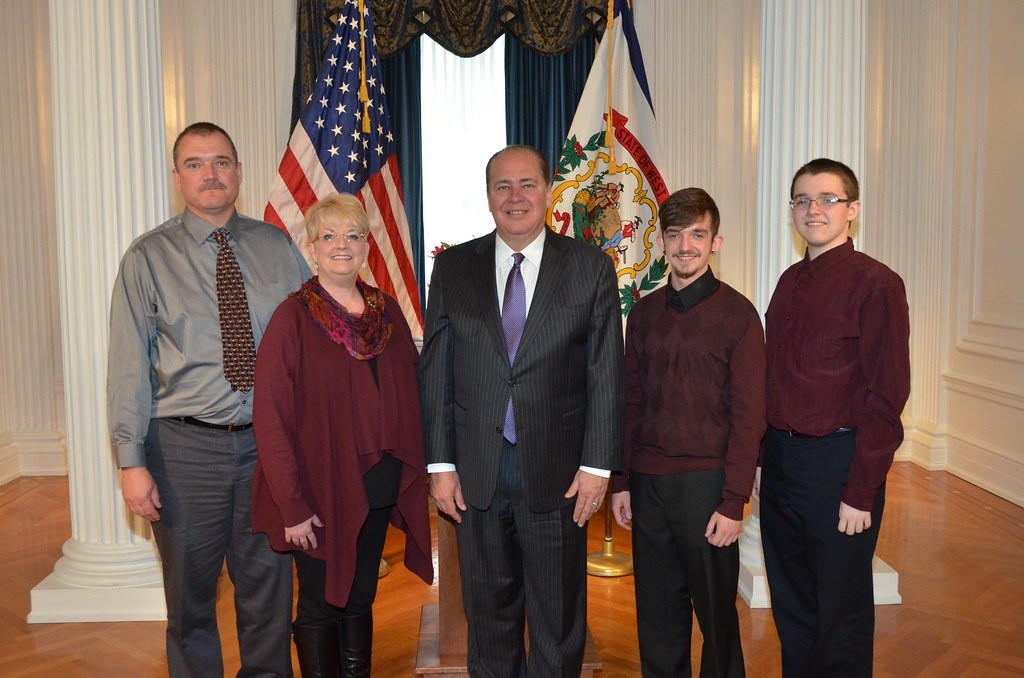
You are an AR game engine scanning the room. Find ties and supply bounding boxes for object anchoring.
[210,228,257,394]
[502,253,526,444]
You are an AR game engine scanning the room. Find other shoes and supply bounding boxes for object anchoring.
[377,558,389,578]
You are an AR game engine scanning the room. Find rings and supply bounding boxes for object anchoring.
[593,503,597,505]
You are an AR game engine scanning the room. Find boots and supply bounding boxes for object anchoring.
[338,606,373,678]
[291,619,338,678]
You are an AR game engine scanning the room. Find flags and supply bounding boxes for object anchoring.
[263,0,428,359]
[545,0,676,336]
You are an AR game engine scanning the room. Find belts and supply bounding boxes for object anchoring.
[774,423,856,439]
[167,414,254,432]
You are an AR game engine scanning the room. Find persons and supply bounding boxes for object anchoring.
[610,186,767,677]
[106,120,306,677]
[250,193,435,678]
[420,148,623,678]
[758,158,910,678]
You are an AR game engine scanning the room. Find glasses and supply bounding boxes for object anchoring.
[789,195,857,209]
[320,231,367,246]
